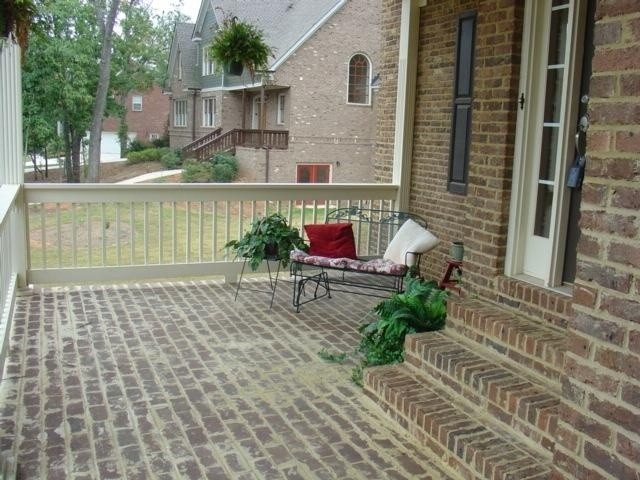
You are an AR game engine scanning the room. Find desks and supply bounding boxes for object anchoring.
[438,260,463,295]
[233,254,306,309]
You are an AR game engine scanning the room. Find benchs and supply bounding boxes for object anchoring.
[290,208,427,313]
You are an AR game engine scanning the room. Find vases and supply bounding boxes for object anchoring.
[451,241,463,261]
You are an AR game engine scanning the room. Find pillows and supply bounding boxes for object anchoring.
[304,223,358,260]
[383,218,441,269]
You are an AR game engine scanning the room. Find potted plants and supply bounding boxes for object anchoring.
[202,6,280,88]
[216,211,310,273]
